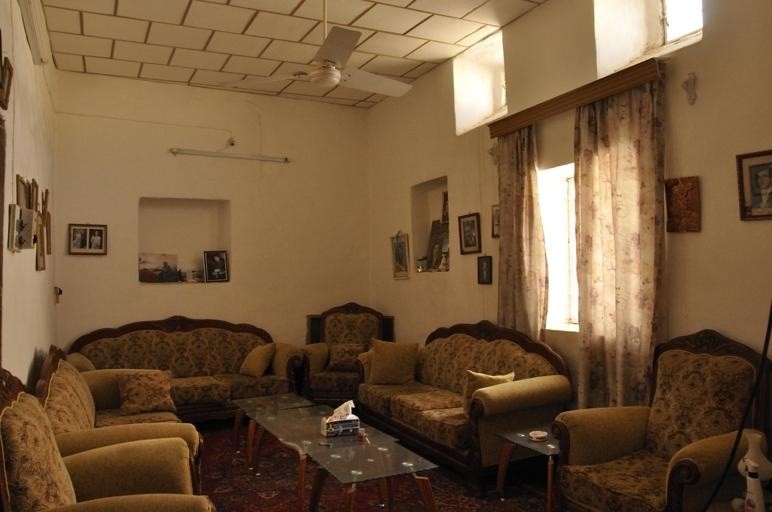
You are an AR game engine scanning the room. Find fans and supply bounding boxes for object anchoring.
[217,0,414,98]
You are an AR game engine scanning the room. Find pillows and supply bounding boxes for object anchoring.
[464,369,515,417]
[238,343,277,379]
[326,343,365,371]
[116,370,177,414]
[368,337,420,385]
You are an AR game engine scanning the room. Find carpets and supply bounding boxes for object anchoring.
[169,399,593,512]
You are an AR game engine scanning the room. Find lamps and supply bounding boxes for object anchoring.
[169,148,289,163]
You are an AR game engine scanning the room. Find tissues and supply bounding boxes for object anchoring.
[320,400,360,437]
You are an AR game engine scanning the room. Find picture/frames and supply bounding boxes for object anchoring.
[16,173,29,207]
[42,188,50,225]
[734,149,772,221]
[1,57,12,113]
[491,204,502,237]
[457,213,481,255]
[35,224,46,271]
[29,178,40,210]
[390,234,410,280]
[138,253,178,283]
[46,212,51,254]
[663,175,702,233]
[203,250,228,282]
[68,223,107,255]
[477,256,493,284]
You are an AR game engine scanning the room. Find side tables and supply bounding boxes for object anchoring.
[496,427,560,511]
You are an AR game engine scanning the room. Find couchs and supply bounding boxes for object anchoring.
[299,301,395,398]
[35,344,203,495]
[0,367,211,512]
[552,327,771,512]
[64,314,299,410]
[354,320,573,483]
